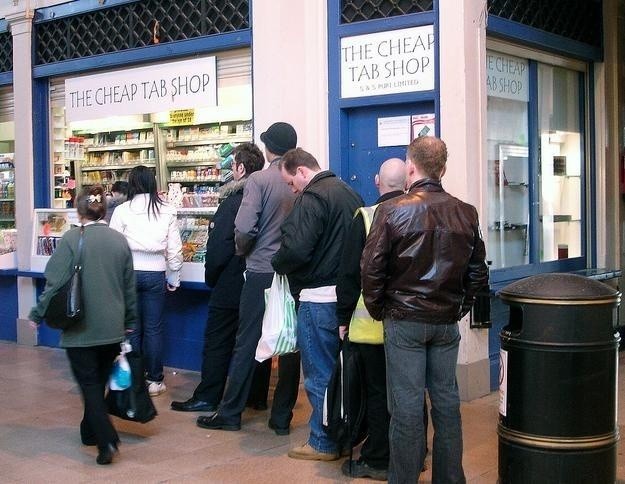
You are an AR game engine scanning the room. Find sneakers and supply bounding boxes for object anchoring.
[288,442,390,480]
[147,381,166,396]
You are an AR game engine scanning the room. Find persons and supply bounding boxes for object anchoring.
[269,145,365,461]
[172,142,273,411]
[360,133,491,483]
[103,181,128,225]
[333,157,429,481]
[28,184,137,465]
[108,165,181,397]
[195,121,301,436]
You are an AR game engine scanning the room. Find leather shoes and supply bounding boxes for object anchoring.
[96,444,117,465]
[170,397,291,435]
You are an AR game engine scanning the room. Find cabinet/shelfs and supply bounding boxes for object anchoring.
[1,140,19,254]
[75,126,249,207]
[52,107,66,207]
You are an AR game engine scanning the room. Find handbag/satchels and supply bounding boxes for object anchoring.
[106,356,157,423]
[45,225,84,330]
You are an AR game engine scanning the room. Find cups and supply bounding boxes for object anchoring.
[52,217,66,232]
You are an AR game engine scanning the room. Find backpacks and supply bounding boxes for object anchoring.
[322,332,368,476]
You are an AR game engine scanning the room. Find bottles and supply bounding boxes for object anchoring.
[0,181,17,255]
[64,137,84,158]
[219,154,235,170]
[218,142,236,158]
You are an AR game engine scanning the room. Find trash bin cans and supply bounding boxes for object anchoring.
[498,273,622,484]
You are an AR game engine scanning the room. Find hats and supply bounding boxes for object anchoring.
[260,122,297,155]
